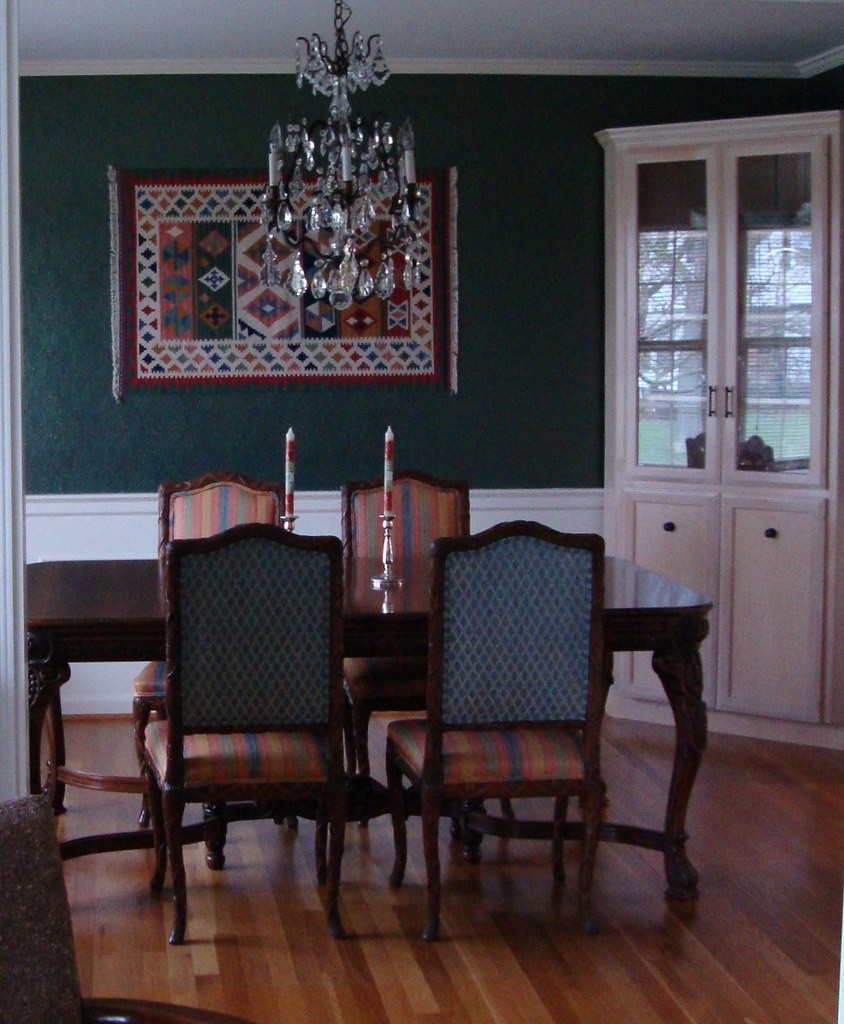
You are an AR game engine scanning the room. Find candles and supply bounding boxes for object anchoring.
[285,427,295,517]
[383,426,393,517]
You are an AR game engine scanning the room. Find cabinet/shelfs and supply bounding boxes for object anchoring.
[592,111,840,751]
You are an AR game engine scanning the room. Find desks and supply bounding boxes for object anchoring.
[23,560,713,899]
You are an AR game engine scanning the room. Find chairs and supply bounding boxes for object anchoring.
[340,471,471,824]
[134,472,292,824]
[142,523,344,943]
[0,797,250,1024]
[386,520,603,940]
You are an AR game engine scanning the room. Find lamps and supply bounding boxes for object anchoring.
[263,42,415,309]
